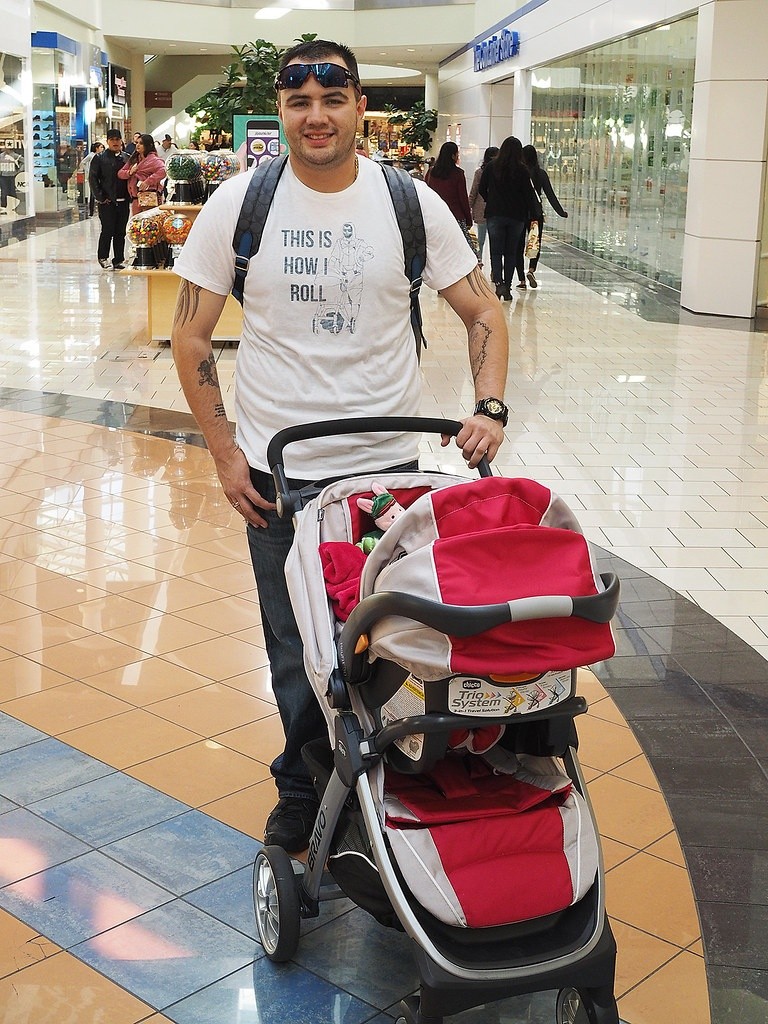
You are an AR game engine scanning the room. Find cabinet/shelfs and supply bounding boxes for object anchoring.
[120,202,244,346]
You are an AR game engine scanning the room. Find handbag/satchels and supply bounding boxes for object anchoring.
[525,224,539,258]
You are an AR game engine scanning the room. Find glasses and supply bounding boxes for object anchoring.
[275,64,360,89]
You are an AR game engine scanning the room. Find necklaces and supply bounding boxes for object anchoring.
[355,155,359,180]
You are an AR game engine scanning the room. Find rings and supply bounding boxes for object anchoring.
[232,502,239,508]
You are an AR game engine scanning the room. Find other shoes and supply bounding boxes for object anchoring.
[504,290,513,300]
[495,282,507,299]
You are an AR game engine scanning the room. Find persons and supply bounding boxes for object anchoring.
[80,128,212,271]
[170,39,509,854]
[355,143,416,159]
[424,142,473,230]
[0,148,24,214]
[41,140,76,193]
[467,135,569,301]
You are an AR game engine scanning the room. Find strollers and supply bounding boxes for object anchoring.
[250,417,621,1024]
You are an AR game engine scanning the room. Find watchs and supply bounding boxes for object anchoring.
[474,396,508,428]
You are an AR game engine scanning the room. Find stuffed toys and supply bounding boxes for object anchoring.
[353,481,405,553]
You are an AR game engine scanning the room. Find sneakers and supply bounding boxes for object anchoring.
[98,258,113,270]
[516,284,526,290]
[264,797,319,852]
[114,265,125,270]
[526,271,537,288]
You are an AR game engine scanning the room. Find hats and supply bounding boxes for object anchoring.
[107,129,121,140]
[162,133,174,141]
